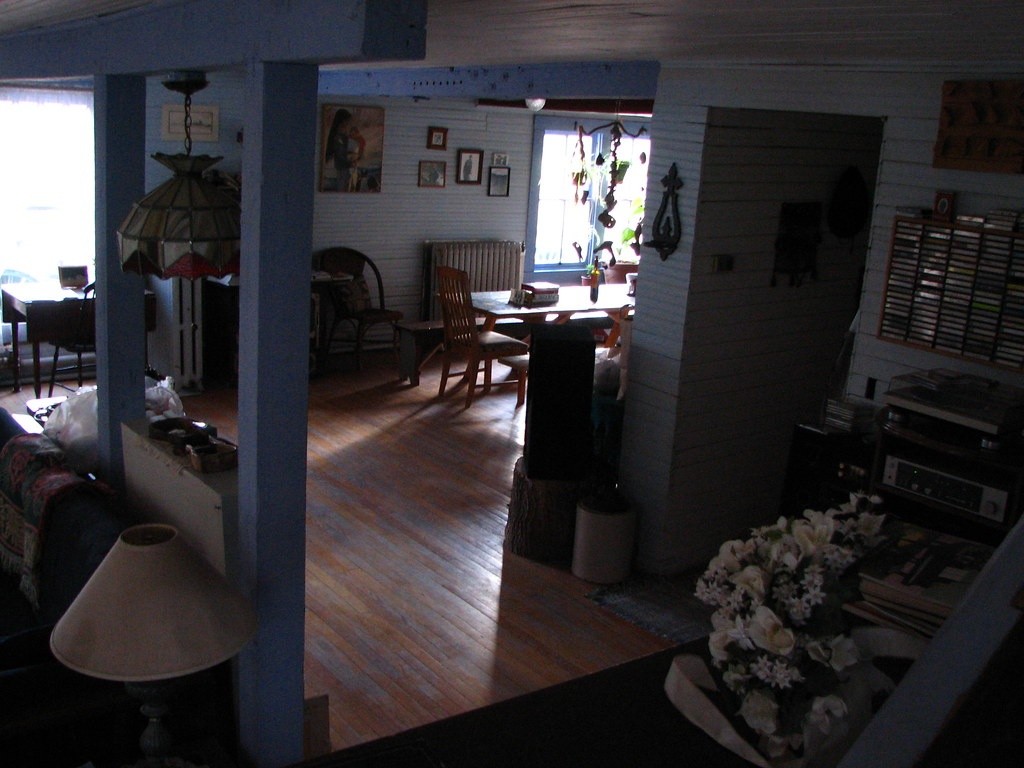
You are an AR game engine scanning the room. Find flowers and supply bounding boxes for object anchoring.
[694,489,887,768]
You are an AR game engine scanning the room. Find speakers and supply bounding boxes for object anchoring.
[778,423,878,521]
[524,323,596,482]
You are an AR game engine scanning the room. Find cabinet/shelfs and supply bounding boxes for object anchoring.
[866,408,1024,549]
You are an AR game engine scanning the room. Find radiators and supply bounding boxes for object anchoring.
[420,239,525,321]
[146,274,206,397]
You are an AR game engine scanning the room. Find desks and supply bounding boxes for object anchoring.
[436,284,635,392]
[1,283,156,399]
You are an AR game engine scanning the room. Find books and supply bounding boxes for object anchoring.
[840,523,997,640]
[520,280,560,303]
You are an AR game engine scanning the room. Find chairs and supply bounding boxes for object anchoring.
[49,282,96,397]
[312,245,405,371]
[437,265,529,409]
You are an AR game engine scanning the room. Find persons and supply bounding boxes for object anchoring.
[464,154,473,181]
[496,155,503,165]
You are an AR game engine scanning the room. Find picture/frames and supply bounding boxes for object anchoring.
[58,266,88,287]
[456,149,484,185]
[319,102,384,192]
[427,127,448,149]
[493,153,508,166]
[489,167,511,197]
[418,160,446,188]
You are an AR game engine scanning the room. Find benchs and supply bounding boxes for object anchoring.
[393,309,635,386]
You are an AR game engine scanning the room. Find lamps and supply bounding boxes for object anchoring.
[50,523,260,768]
[116,72,240,281]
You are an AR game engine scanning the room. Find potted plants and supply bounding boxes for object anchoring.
[581,264,596,286]
[603,197,645,284]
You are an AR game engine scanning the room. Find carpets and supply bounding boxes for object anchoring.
[584,562,721,644]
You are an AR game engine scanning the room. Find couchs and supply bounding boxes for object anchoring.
[0,408,239,768]
